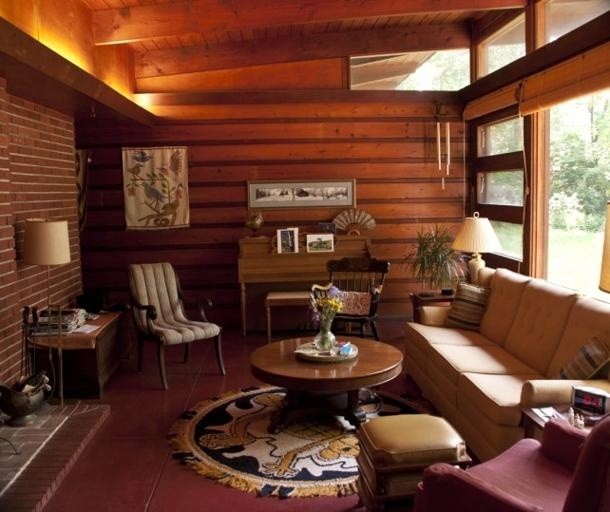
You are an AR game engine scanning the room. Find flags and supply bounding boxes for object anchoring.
[314,319,336,351]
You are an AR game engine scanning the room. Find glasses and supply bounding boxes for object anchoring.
[23,217,71,409]
[451,212,504,286]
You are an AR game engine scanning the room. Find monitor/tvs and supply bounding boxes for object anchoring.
[28,306,89,337]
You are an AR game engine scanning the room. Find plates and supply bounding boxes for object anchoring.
[29,308,131,400]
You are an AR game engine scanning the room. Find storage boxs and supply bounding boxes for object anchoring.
[263,291,367,344]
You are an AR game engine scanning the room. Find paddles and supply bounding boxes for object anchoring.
[167,384,430,499]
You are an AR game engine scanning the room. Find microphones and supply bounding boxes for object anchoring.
[355,414,472,512]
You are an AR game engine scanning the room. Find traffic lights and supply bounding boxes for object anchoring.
[445,268,610,380]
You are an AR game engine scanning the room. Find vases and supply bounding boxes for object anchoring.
[309,286,347,351]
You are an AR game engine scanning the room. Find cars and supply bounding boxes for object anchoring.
[442,289,452,295]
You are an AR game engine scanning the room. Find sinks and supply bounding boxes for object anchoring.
[405,268,610,464]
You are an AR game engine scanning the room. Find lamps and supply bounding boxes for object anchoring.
[127,262,226,390]
[417,412,610,512]
[319,257,391,341]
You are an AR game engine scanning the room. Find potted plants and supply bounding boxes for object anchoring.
[276,228,299,254]
[246,177,357,210]
[306,234,334,252]
